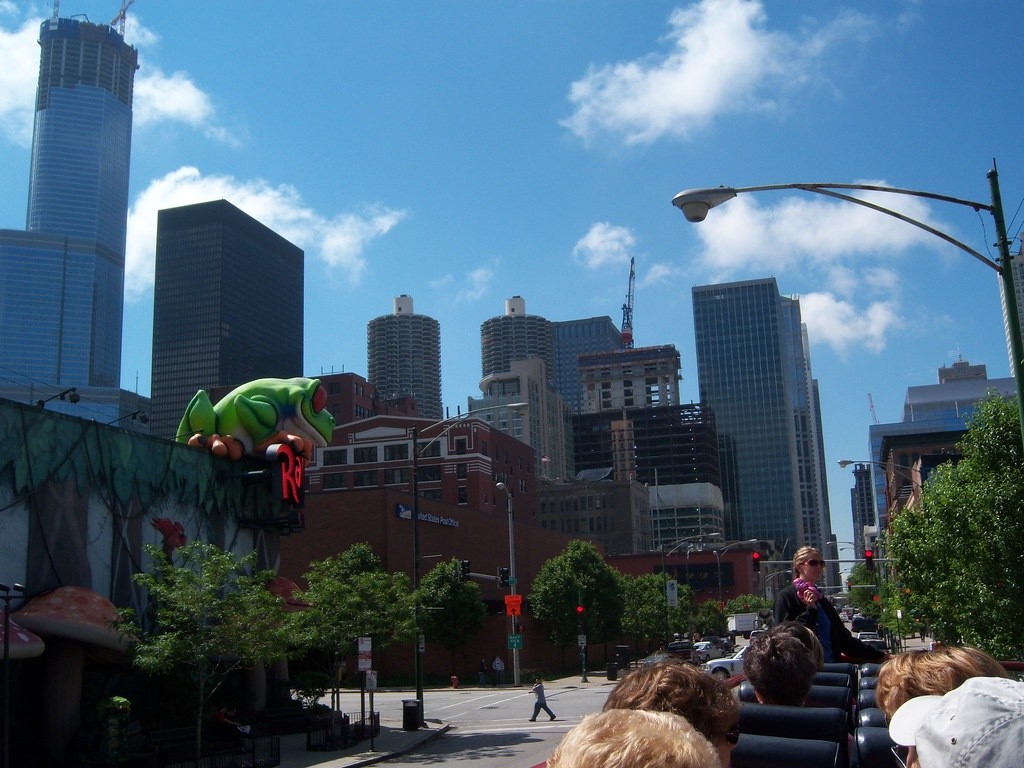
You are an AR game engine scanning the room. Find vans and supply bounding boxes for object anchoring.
[668,641,701,666]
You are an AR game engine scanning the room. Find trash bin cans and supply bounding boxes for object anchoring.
[607,662,618,680]
[615,646,630,670]
[402,699,421,731]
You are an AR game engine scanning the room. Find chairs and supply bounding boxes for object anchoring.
[727,661,909,768]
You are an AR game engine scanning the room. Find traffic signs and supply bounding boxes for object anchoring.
[578,634,586,646]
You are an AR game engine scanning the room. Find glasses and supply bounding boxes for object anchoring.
[798,560,826,568]
[884,714,891,726]
[710,723,741,745]
[891,745,907,768]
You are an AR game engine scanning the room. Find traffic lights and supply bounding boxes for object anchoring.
[458,559,471,582]
[517,622,526,633]
[865,549,874,570]
[752,550,760,572]
[577,604,585,634]
[497,566,509,590]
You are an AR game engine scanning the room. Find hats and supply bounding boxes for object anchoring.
[889,677,1024,768]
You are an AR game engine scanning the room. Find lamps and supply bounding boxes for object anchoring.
[35,387,80,406]
[169,428,208,443]
[106,409,149,425]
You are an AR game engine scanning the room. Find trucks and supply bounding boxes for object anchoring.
[728,613,758,635]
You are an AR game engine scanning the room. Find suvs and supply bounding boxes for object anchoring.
[702,646,747,680]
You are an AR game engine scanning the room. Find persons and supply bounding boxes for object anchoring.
[492,655,505,686]
[586,545,1024,768]
[529,678,556,721]
[208,703,249,755]
[478,657,488,686]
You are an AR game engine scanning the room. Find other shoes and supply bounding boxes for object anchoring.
[550,715,556,721]
[529,719,536,722]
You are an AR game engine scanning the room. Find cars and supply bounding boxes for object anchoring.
[693,635,735,661]
[839,604,865,631]
[857,632,886,649]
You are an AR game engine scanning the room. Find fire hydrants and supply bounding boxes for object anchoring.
[450,676,458,688]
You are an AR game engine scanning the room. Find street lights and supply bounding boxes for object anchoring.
[413,403,529,723]
[759,569,792,600]
[660,533,721,649]
[671,157,1024,451]
[496,482,521,686]
[826,540,896,656]
[713,538,757,613]
